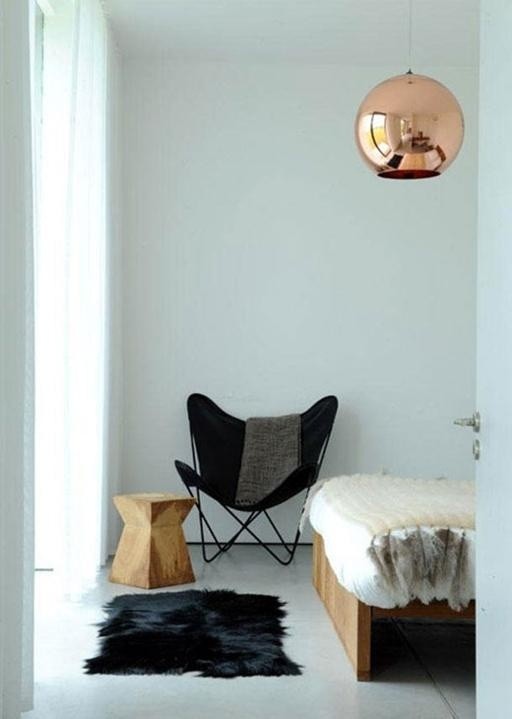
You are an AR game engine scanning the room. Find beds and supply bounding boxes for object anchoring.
[382,145,446,171]
[297,475,476,680]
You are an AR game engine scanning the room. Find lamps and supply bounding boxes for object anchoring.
[355,0,466,182]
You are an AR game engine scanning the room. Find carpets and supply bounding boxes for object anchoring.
[82,591,304,678]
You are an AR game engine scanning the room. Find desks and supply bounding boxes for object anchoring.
[107,493,196,589]
[410,137,430,150]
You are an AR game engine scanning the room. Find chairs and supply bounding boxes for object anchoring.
[173,393,339,566]
